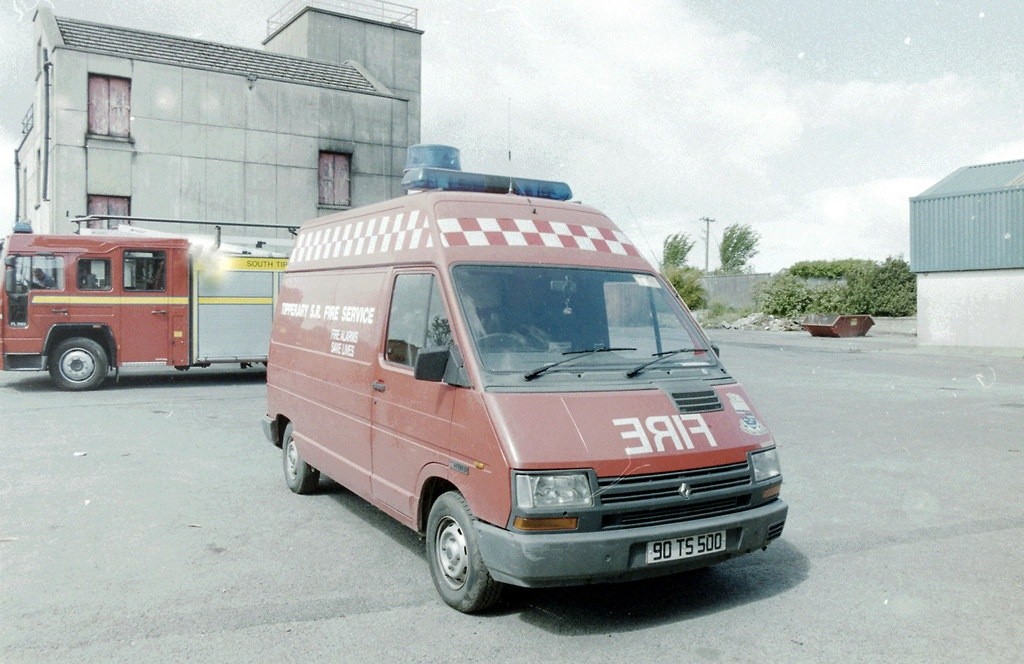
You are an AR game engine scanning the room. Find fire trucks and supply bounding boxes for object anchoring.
[0,213,303,392]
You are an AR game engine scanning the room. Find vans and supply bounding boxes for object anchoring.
[259,143,790,615]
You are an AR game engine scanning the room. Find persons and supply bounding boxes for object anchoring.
[33,268,55,288]
